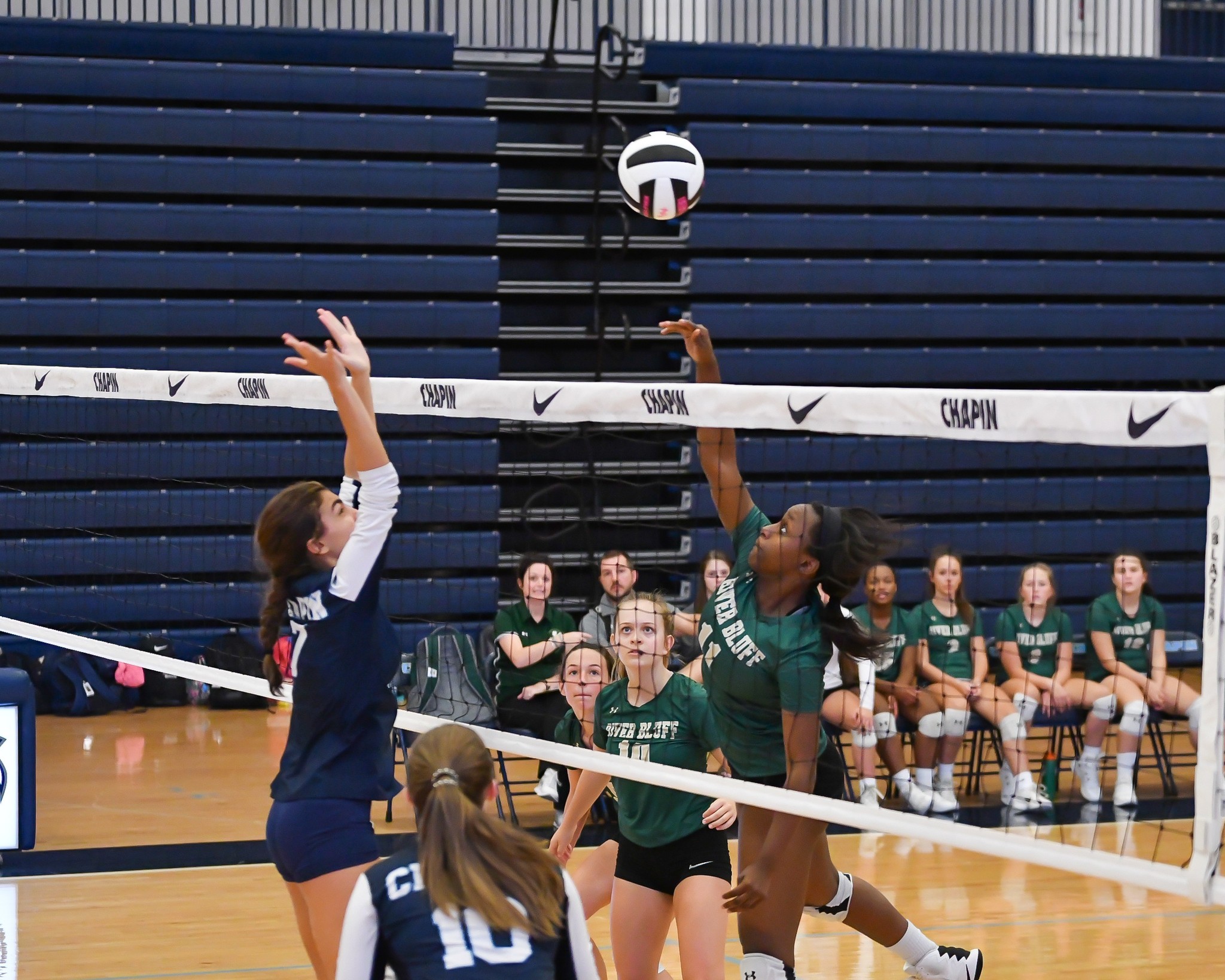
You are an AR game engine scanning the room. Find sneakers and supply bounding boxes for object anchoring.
[552,811,564,829]
[903,945,983,980]
[533,768,562,802]
[860,786,885,808]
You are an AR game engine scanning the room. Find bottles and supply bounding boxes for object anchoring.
[1039,749,1057,801]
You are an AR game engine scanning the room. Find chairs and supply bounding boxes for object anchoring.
[383,628,1203,828]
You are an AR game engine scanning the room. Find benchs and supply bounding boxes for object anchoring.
[629,37,1225,668]
[0,15,503,676]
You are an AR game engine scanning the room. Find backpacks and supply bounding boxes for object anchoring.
[207,635,268,710]
[0,635,187,717]
[404,626,497,724]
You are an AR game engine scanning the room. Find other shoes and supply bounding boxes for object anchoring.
[907,784,955,812]
[936,781,960,811]
[1112,778,1138,806]
[1070,751,1104,802]
[900,782,932,814]
[1010,770,1054,810]
[999,770,1040,810]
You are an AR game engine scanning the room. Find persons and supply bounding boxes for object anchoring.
[817,583,881,807]
[493,551,591,833]
[839,561,943,814]
[660,316,986,980]
[989,562,1118,801]
[577,550,734,677]
[903,546,1053,811]
[334,722,609,980]
[792,802,1145,979]
[256,307,404,980]
[1082,550,1202,807]
[548,592,738,980]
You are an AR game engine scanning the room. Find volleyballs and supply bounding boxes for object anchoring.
[617,131,705,221]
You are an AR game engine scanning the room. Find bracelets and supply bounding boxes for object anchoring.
[541,680,550,691]
[548,636,563,650]
